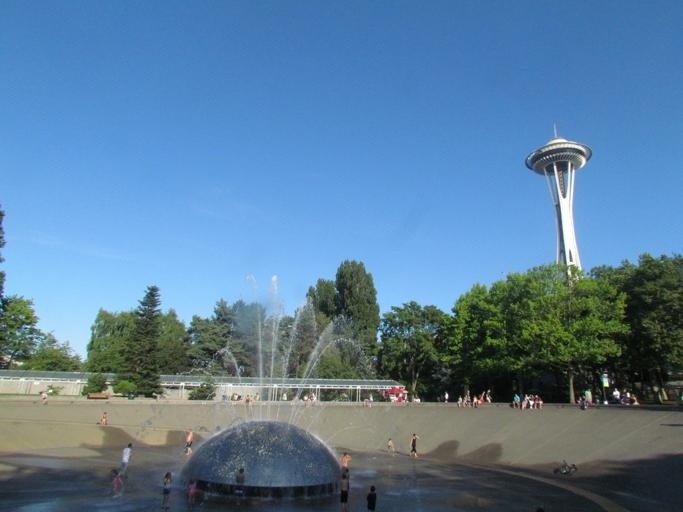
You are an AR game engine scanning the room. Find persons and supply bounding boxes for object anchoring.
[343,453,352,479]
[184,431,193,455]
[367,486,376,512]
[340,474,349,512]
[39,391,48,406]
[111,469,123,499]
[121,443,132,481]
[561,459,570,481]
[457,389,492,409]
[387,439,394,457]
[369,393,373,402]
[437,391,449,404]
[577,387,640,411]
[512,393,545,411]
[410,434,419,458]
[222,393,259,403]
[188,479,197,505]
[161,472,171,510]
[282,391,287,400]
[236,468,244,505]
[303,392,316,408]
[101,412,109,425]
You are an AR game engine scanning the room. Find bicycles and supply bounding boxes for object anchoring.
[553,460,577,475]
